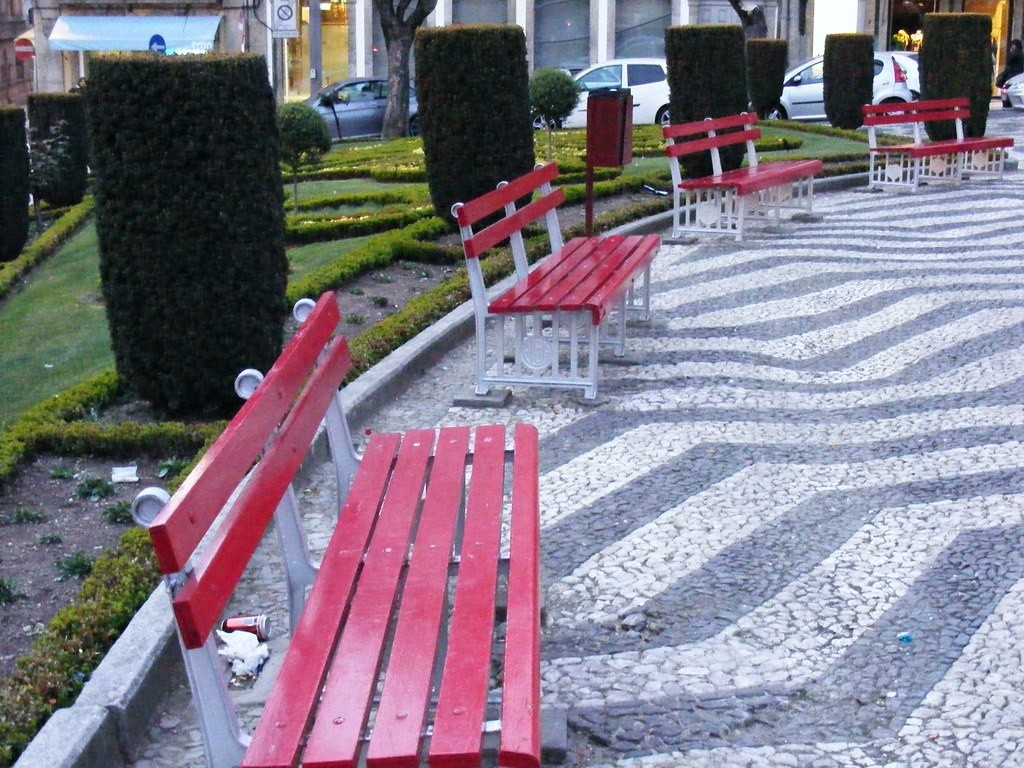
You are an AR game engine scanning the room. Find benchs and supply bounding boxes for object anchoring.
[863,97,1014,195]
[662,111,824,241]
[130,290,568,767]
[450,161,663,404]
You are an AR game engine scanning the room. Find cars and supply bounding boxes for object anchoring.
[528,57,672,130]
[760,54,913,121]
[302,76,420,146]
[999,72,1024,111]
[810,51,921,98]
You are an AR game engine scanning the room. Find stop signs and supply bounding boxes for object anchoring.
[15,38,34,60]
